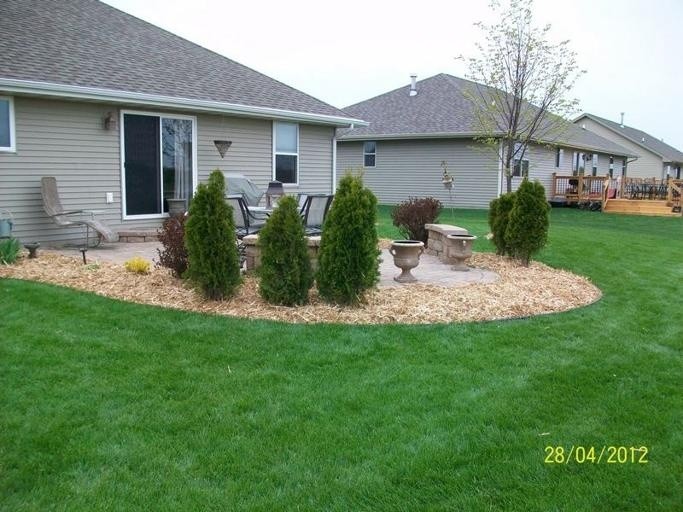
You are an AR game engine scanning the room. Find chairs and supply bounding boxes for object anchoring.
[224,191,270,268]
[39,177,120,249]
[165,199,189,218]
[624,183,667,200]
[295,193,334,236]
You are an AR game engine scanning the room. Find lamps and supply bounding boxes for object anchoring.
[104,111,118,131]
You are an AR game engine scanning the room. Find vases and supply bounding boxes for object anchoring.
[446,232,477,271]
[388,240,424,283]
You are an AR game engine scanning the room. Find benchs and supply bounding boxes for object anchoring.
[242,233,322,270]
[424,223,468,264]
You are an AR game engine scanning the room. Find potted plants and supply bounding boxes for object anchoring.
[569,179,578,194]
